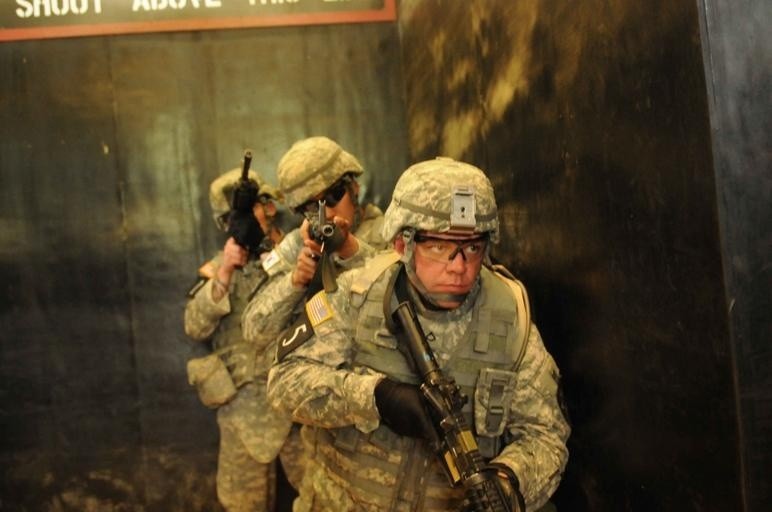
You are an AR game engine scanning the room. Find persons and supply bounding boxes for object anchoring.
[184,163,308,511]
[264,155,572,511]
[244,137,397,493]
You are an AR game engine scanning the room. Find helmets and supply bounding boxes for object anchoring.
[275,134,366,215]
[208,167,275,217]
[379,155,503,245]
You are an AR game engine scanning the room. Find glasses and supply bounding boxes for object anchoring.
[297,179,350,216]
[410,236,490,264]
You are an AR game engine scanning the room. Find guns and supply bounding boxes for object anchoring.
[392,302,513,512]
[230,151,259,272]
[302,199,333,247]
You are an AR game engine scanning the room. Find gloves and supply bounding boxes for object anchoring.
[372,376,449,452]
